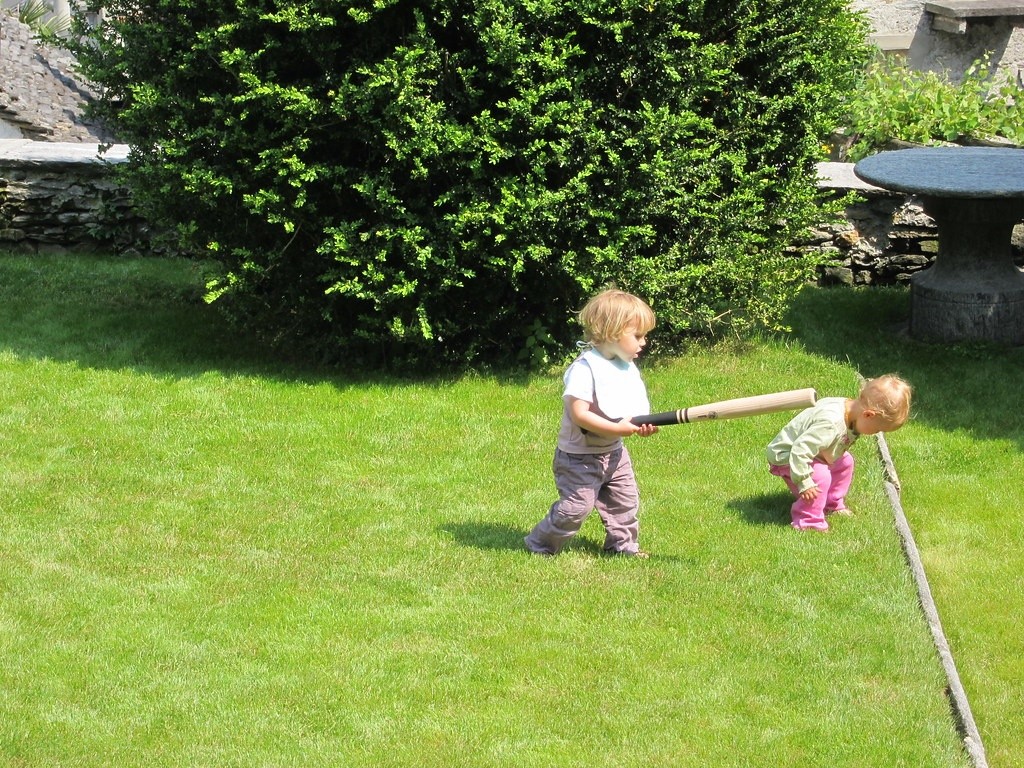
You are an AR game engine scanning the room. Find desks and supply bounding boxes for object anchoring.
[853,147,1024,345]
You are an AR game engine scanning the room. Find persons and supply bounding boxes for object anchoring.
[765,372,919,531]
[520,291,663,555]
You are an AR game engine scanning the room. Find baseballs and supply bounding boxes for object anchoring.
[580,387,817,434]
